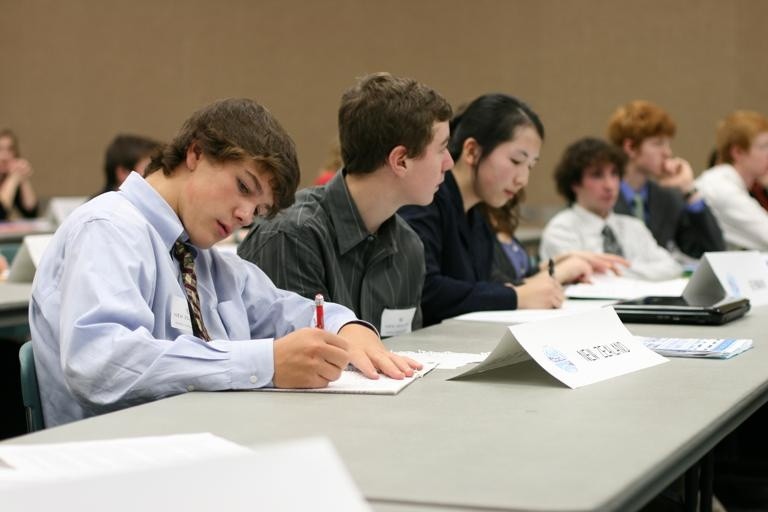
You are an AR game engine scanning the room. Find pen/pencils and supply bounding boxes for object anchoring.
[549,258,555,276]
[315,293,325,329]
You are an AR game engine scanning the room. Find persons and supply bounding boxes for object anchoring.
[607,101,727,261]
[698,107,768,254]
[492,186,630,289]
[537,136,684,282]
[239,70,453,333]
[396,91,563,329]
[28,96,423,428]
[92,132,161,196]
[0,129,39,223]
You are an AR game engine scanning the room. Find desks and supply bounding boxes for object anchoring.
[0,225,766,511]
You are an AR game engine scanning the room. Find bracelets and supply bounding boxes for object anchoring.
[681,188,699,201]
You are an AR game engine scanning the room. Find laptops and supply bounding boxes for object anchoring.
[611,292,750,326]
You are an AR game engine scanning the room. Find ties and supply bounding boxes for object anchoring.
[175,240,211,342]
[633,195,644,223]
[602,226,623,256]
